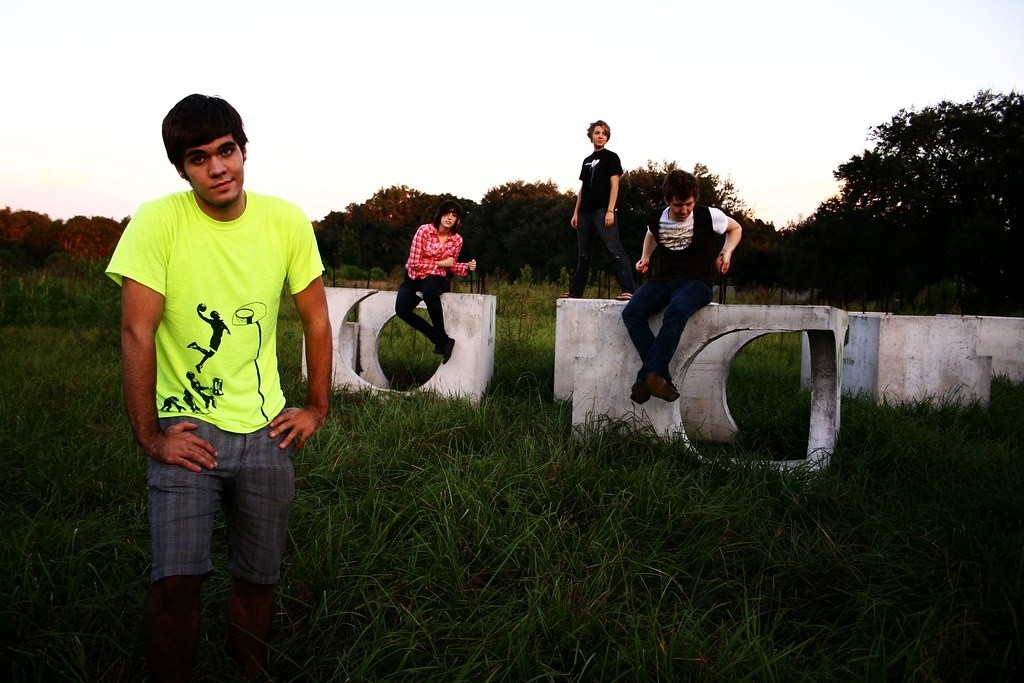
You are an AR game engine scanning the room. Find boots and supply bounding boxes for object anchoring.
[432,335,450,355]
[439,335,455,366]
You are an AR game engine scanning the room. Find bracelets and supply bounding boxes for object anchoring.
[606,210,614,213]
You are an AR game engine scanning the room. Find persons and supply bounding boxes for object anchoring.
[102,93,331,682]
[562,120,635,301]
[622,170,742,404]
[394,200,477,365]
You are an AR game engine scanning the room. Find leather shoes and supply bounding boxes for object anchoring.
[646,373,680,402]
[630,382,650,404]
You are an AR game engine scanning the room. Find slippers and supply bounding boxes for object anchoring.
[615,292,633,300]
[559,291,582,298]
